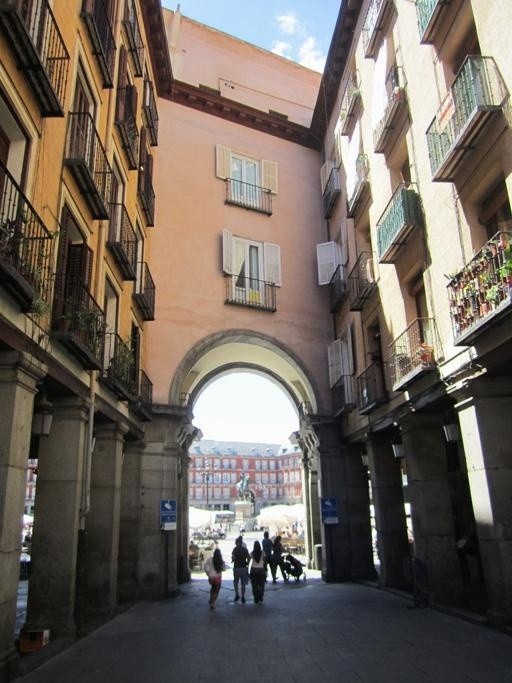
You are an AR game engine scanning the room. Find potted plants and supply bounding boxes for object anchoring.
[54,303,95,342]
[447,231,512,332]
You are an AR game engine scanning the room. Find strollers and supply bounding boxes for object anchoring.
[280,549,307,582]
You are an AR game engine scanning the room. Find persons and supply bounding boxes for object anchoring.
[189,541,200,569]
[247,541,268,604]
[273,534,287,582]
[231,536,250,603]
[261,531,276,583]
[282,520,303,538]
[206,519,269,543]
[204,548,226,609]
[285,554,306,581]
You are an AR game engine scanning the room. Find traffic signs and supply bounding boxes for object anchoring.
[159,499,177,531]
